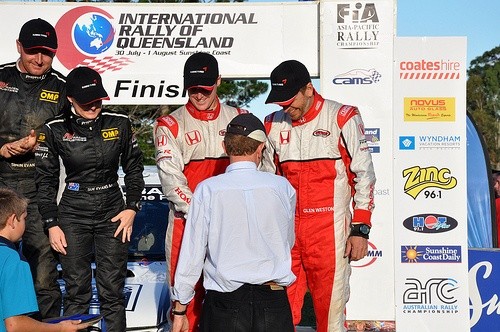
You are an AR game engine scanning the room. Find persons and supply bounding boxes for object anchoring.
[170,112,297,332]
[0,18,68,322]
[258,59,376,332]
[0,187,89,332]
[494,171,500,248]
[153,52,249,332]
[34,67,145,332]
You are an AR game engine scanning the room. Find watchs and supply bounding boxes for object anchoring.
[172,309,185,315]
[130,201,142,211]
[352,224,370,234]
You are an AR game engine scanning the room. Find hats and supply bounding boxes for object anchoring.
[226,113,267,144]
[66,67,110,106]
[184,53,218,92]
[19,18,57,53]
[265,60,311,106]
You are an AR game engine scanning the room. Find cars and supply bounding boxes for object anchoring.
[57,165,171,332]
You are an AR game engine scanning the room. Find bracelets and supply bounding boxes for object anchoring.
[350,231,369,239]
